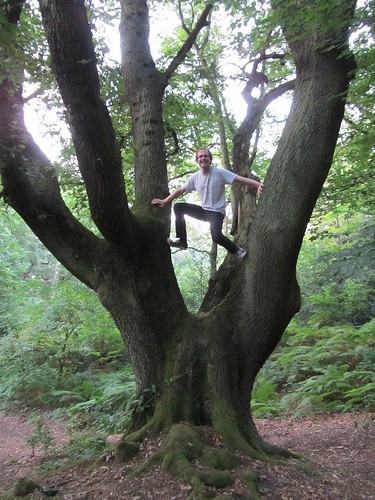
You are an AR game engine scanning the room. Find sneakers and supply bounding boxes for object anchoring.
[237,246,246,258]
[167,239,187,250]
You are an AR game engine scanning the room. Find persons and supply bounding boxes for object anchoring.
[151,148,264,262]
